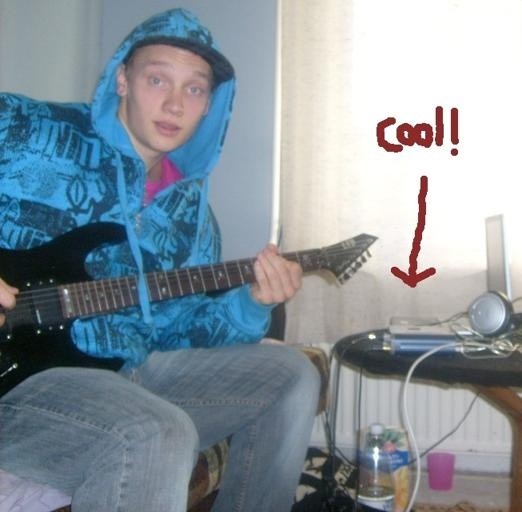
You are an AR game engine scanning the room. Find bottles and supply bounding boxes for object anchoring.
[353,423,395,512]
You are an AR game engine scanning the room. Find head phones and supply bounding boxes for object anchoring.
[468,216,513,336]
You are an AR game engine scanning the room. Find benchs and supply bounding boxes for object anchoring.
[0,339,333,512]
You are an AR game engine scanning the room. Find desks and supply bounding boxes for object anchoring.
[336,330,521,510]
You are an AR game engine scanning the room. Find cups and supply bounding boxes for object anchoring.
[427,452,457,493]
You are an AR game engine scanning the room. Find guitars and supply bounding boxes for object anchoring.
[0,222,378,396]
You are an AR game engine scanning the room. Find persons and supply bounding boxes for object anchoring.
[0,6,323,511]
